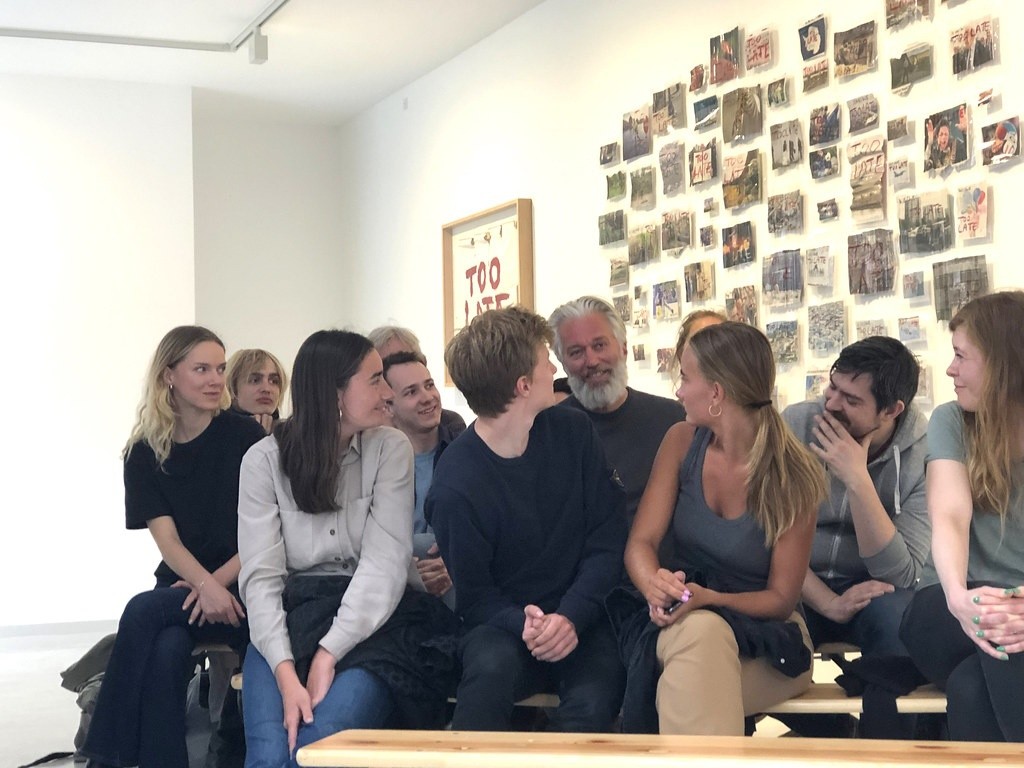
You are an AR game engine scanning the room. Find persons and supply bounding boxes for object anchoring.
[601,21,1018,371]
[675,310,728,360]
[780,336,931,739]
[237,330,412,768]
[78,327,269,768]
[225,348,287,434]
[370,326,466,613]
[622,321,823,736]
[423,305,628,733]
[551,296,686,524]
[899,291,1024,741]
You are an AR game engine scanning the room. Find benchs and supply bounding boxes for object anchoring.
[296,728,1024,768]
[445,682,947,717]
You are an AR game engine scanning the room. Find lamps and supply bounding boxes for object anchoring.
[249,26,269,63]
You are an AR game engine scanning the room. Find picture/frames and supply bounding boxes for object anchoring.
[441,199,536,386]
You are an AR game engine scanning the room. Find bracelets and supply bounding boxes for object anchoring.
[196,574,210,591]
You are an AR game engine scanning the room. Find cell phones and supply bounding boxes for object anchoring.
[664,592,694,614]
